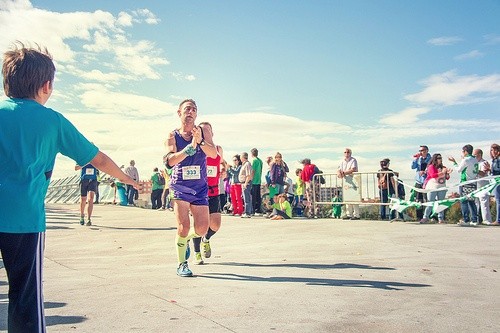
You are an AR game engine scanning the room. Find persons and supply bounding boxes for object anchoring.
[485,144,500,225]
[376,158,405,221]
[75,163,100,226]
[164,99,217,277]
[150,166,175,211]
[411,145,432,220]
[472,149,492,224]
[449,144,479,225]
[0,41,139,333]
[331,190,343,218]
[111,160,139,207]
[419,154,453,223]
[192,122,224,265]
[336,148,360,219]
[218,148,321,220]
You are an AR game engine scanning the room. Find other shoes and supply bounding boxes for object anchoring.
[487,221,500,226]
[482,221,491,225]
[270,215,281,220]
[420,218,430,224]
[80,214,84,225]
[129,204,134,207]
[241,213,251,218]
[457,221,477,226]
[438,220,444,224]
[158,207,165,211]
[343,215,360,220]
[86,219,91,226]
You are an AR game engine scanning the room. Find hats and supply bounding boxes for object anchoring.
[240,152,248,158]
[251,148,258,156]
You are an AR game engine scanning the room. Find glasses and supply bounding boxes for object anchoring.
[437,157,441,159]
[344,152,349,153]
[419,150,424,152]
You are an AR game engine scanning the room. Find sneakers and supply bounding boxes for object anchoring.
[194,252,204,265]
[185,246,190,260]
[177,261,192,276]
[202,241,211,258]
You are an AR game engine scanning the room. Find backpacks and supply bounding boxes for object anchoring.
[155,173,165,185]
[378,170,387,189]
